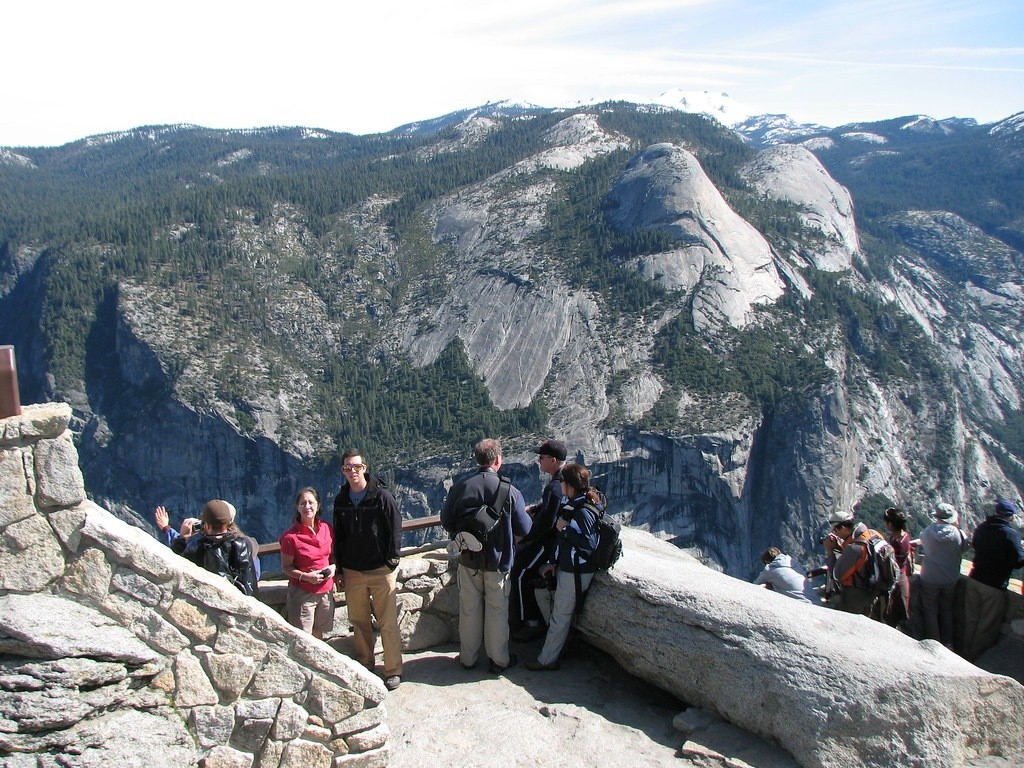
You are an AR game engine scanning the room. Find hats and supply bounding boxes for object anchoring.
[534,440,567,461]
[198,500,232,524]
[222,500,236,520]
[935,503,959,524]
[996,500,1016,516]
[828,511,854,534]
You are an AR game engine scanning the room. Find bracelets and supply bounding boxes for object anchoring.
[299,572,305,583]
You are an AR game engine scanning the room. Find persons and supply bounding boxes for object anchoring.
[881,507,913,631]
[962,499,1024,663]
[278,488,338,641]
[753,546,824,607]
[332,447,402,691]
[524,464,606,670]
[509,440,570,644]
[819,510,883,621]
[154,500,261,597]
[439,439,532,674]
[919,503,969,652]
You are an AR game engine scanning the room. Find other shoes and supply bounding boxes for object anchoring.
[489,655,517,673]
[385,675,401,689]
[565,629,577,642]
[525,658,560,671]
[462,663,475,670]
[512,619,546,643]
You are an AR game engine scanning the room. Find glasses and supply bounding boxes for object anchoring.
[829,520,842,525]
[343,465,363,472]
[299,501,317,509]
[559,476,567,483]
[884,506,896,520]
[539,456,553,460]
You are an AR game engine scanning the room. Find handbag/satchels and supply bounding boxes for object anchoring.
[906,548,915,575]
[456,504,503,572]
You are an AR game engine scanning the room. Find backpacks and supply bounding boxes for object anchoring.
[843,530,899,624]
[568,503,624,612]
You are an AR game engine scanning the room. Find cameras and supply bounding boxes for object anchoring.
[193,519,202,532]
[819,536,833,544]
